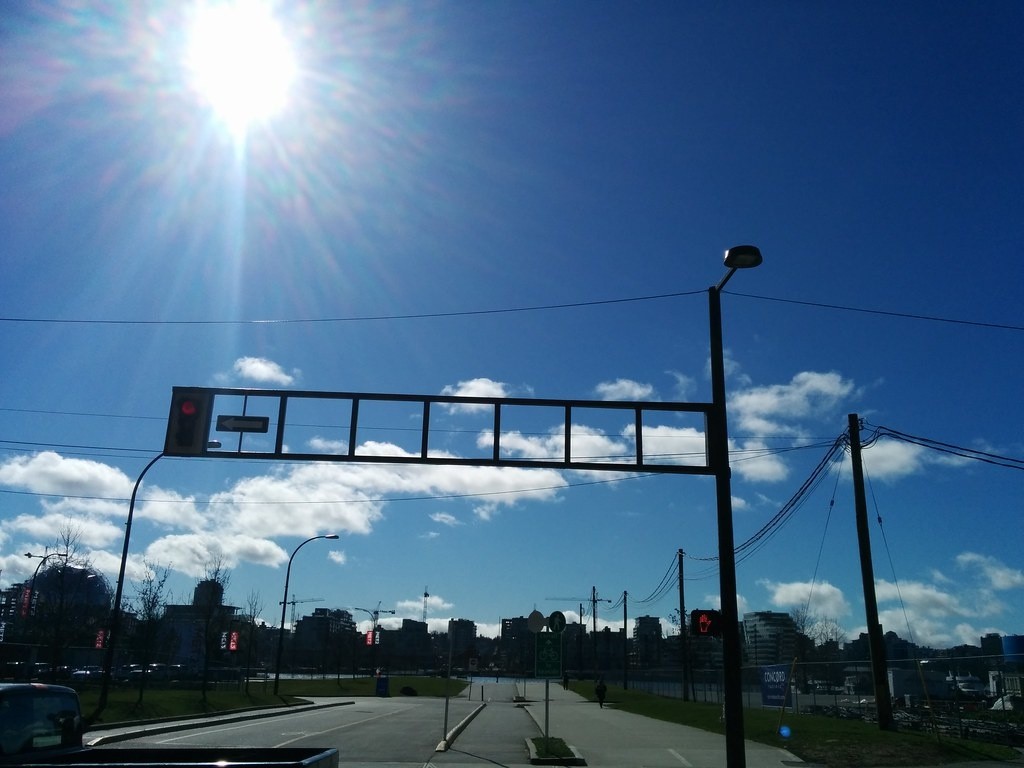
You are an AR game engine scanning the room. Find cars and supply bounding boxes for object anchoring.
[956,682,984,701]
[1,658,196,684]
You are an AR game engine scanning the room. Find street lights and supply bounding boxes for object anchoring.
[20,554,68,642]
[706,244,763,768]
[273,535,338,684]
[96,440,223,706]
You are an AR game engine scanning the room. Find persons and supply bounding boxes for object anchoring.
[560,668,569,690]
[595,680,607,709]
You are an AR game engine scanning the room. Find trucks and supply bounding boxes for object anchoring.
[0,683,340,768]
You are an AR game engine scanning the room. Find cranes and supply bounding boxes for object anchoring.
[278,594,328,635]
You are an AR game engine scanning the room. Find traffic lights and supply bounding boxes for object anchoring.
[164,384,214,458]
[691,610,721,638]
